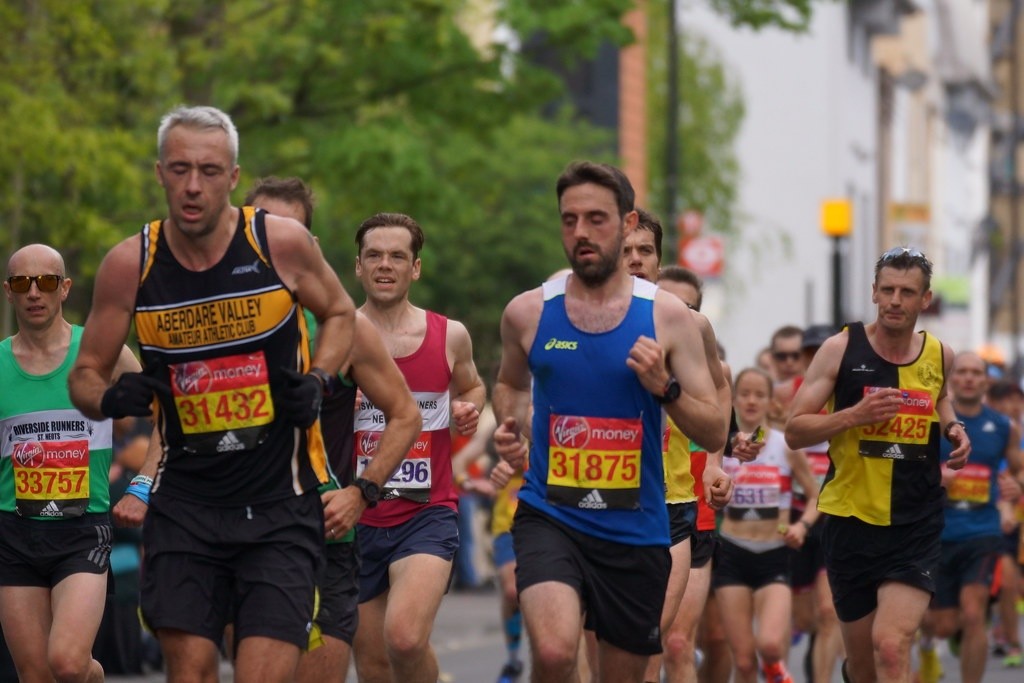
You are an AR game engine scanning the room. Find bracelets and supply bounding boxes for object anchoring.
[124,475,154,506]
[797,519,812,530]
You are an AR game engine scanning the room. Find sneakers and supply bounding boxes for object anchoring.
[916,645,944,682]
[758,657,794,683]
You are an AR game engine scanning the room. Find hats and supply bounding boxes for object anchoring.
[975,343,1003,365]
[800,325,837,348]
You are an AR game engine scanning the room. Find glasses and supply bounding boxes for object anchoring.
[7,273,63,294]
[774,352,801,361]
[883,247,928,264]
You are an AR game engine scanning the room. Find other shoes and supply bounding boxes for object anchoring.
[497,660,523,683]
[1003,647,1023,666]
[948,630,963,659]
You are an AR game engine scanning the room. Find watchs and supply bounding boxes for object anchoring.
[943,420,968,442]
[652,377,682,403]
[309,368,336,396]
[351,478,381,509]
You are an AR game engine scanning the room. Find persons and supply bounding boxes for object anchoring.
[244,174,424,683]
[784,243,972,683]
[0,244,169,683]
[350,213,487,683]
[921,349,1024,683]
[709,366,822,683]
[451,209,1024,683]
[65,103,357,683]
[490,158,727,683]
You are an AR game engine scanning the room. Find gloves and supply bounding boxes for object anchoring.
[100,372,173,421]
[276,366,323,430]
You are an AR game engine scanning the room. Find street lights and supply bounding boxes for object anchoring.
[821,196,858,328]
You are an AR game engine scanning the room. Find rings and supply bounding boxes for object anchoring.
[464,423,468,430]
[329,528,337,536]
